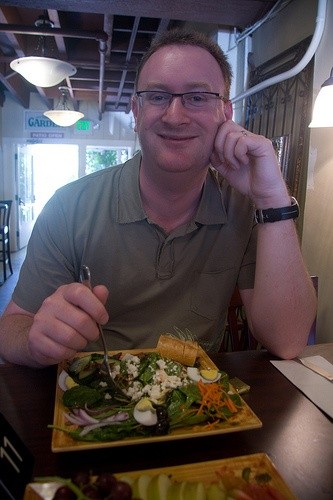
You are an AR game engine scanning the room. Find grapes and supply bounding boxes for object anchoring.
[51,468,140,500]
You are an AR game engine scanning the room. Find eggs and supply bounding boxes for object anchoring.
[133,397,158,425]
[59,370,79,391]
[186,367,222,383]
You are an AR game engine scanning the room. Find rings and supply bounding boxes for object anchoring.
[240,130,248,136]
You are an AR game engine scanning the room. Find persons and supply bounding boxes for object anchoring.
[0,27,316,369]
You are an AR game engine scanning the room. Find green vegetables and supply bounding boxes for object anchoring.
[47,352,242,441]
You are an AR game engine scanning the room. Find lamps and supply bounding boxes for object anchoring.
[43,86,85,127]
[9,18,77,88]
[308,67,333,128]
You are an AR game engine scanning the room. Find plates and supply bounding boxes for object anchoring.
[23,454,297,500]
[52,345,262,451]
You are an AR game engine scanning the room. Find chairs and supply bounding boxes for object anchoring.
[0,200,13,281]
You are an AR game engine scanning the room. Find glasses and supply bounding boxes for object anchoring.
[136,90,230,111]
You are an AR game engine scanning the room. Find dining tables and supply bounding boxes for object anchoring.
[0,340,333,500]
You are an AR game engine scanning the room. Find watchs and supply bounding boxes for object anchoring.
[254,196,300,223]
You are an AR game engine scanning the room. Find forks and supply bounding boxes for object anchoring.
[78,264,133,402]
